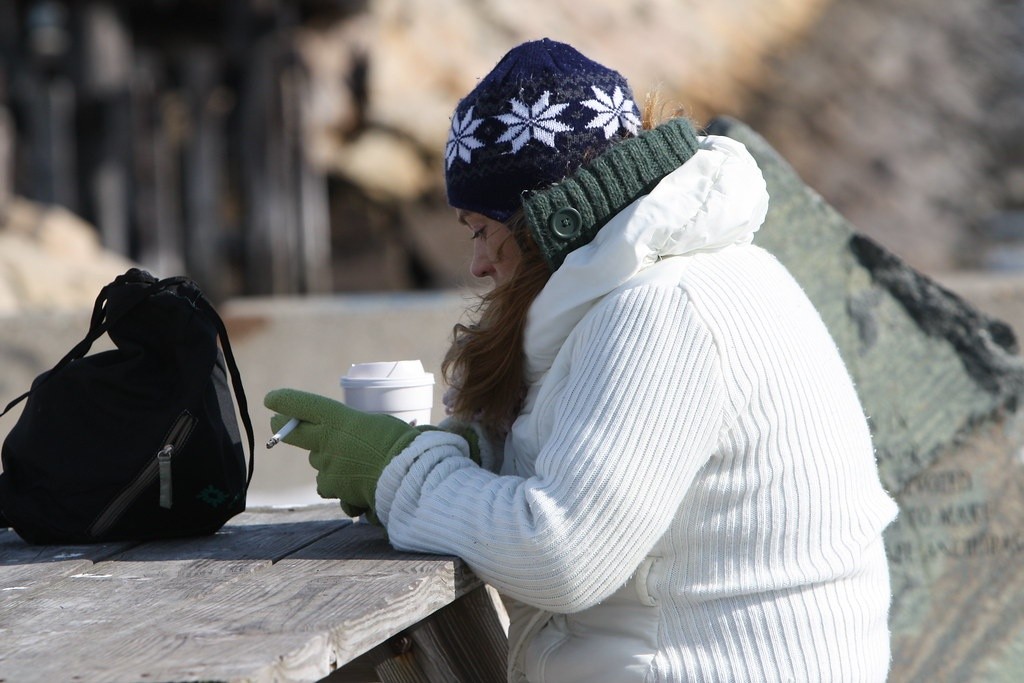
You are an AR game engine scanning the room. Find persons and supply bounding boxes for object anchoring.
[261,35,902,683]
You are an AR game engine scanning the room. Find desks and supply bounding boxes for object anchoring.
[0,502,512,683]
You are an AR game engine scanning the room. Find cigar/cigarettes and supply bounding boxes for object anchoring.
[266,417,300,449]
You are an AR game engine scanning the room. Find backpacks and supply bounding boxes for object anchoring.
[0,267,251,546]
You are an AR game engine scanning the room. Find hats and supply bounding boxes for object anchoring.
[446,40,643,258]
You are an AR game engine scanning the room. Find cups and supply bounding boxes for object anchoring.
[340,361,435,523]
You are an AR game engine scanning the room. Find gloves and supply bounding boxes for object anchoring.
[264,388,417,512]
[342,424,481,526]
